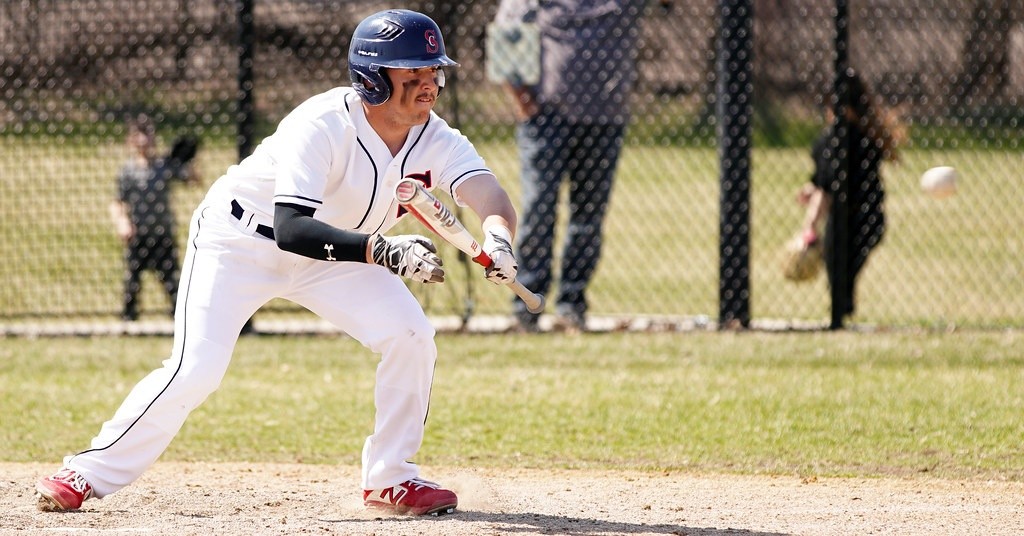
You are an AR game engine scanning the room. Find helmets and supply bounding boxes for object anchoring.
[348,9,461,107]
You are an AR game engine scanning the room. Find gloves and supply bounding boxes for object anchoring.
[372,233,444,285]
[483,226,519,285]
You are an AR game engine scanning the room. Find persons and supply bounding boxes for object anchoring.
[484,0,640,334]
[32,7,521,519]
[108,110,202,321]
[810,72,901,329]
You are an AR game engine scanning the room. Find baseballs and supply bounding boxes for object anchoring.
[920,162,962,200]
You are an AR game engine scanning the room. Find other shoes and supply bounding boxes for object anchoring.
[552,318,586,334]
[504,321,540,333]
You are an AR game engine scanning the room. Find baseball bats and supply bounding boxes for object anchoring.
[393,178,547,317]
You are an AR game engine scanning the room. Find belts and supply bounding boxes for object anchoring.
[231,201,275,240]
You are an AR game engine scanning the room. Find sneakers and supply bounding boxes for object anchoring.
[35,469,96,512]
[363,477,457,519]
